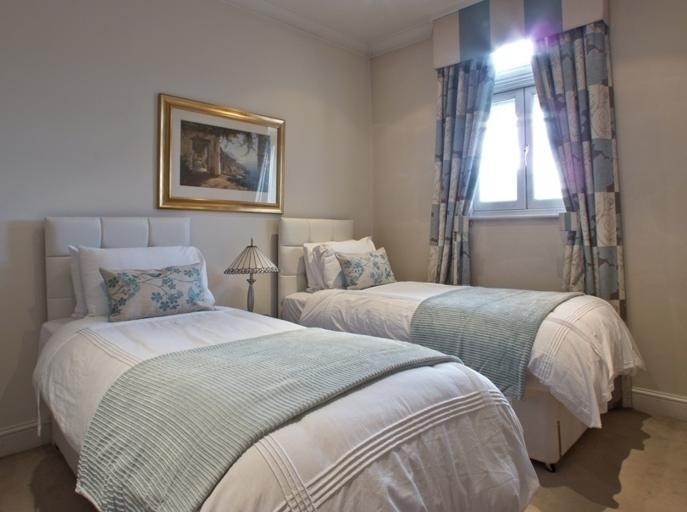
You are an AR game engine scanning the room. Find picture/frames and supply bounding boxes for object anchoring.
[156,93,285,214]
